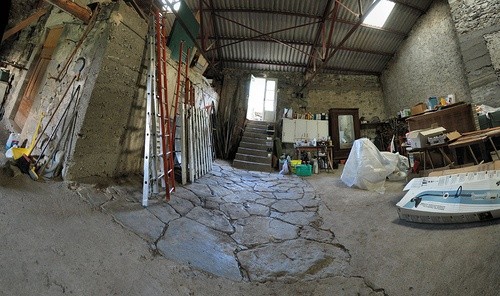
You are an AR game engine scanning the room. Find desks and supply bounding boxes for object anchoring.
[413,129,500,174]
[361,122,389,151]
[296,145,334,170]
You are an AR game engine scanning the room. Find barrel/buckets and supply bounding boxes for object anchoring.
[290,160,302,173]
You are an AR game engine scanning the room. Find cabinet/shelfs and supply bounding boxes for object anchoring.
[281,119,306,143]
[328,107,361,160]
[405,102,479,165]
[307,120,328,138]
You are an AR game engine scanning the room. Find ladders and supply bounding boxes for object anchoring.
[172,41,190,173]
[156,12,176,202]
[143,15,160,209]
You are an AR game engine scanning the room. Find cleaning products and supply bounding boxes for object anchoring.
[5,133,21,150]
[296,161,312,176]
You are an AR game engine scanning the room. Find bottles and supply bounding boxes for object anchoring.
[5,133,20,149]
[296,166,301,176]
[279,156,285,170]
[286,155,291,170]
[294,111,297,119]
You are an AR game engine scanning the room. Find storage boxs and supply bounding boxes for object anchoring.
[412,102,428,115]
[445,130,463,144]
[407,126,447,148]
[400,108,411,118]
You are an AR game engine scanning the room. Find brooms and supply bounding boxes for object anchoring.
[15,75,83,173]
[28,85,83,180]
